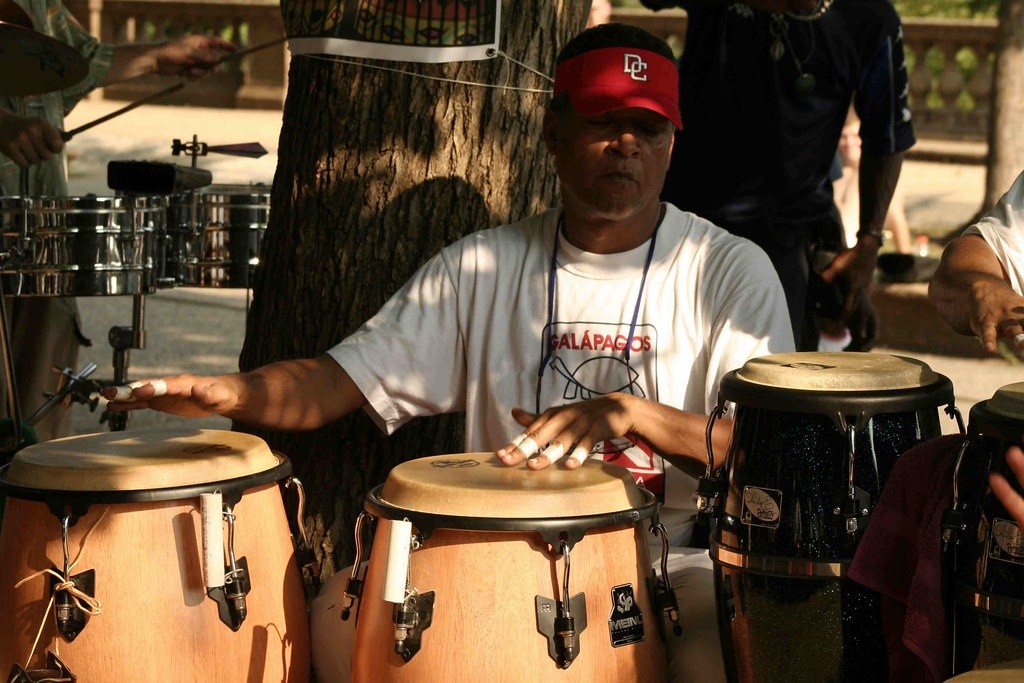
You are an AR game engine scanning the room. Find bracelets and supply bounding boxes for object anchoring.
[855,226,884,248]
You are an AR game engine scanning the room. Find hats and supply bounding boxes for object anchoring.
[552,47,683,130]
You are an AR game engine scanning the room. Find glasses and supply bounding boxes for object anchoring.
[535,350,638,457]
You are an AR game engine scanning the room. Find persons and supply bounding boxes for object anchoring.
[926,170,1024,531]
[661,0,918,351]
[101,23,795,683]
[1,0,238,442]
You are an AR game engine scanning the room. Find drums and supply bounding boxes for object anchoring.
[940,381,1024,671]
[696,351,955,683]
[2,184,274,299]
[339,450,683,683]
[0,427,312,683]
